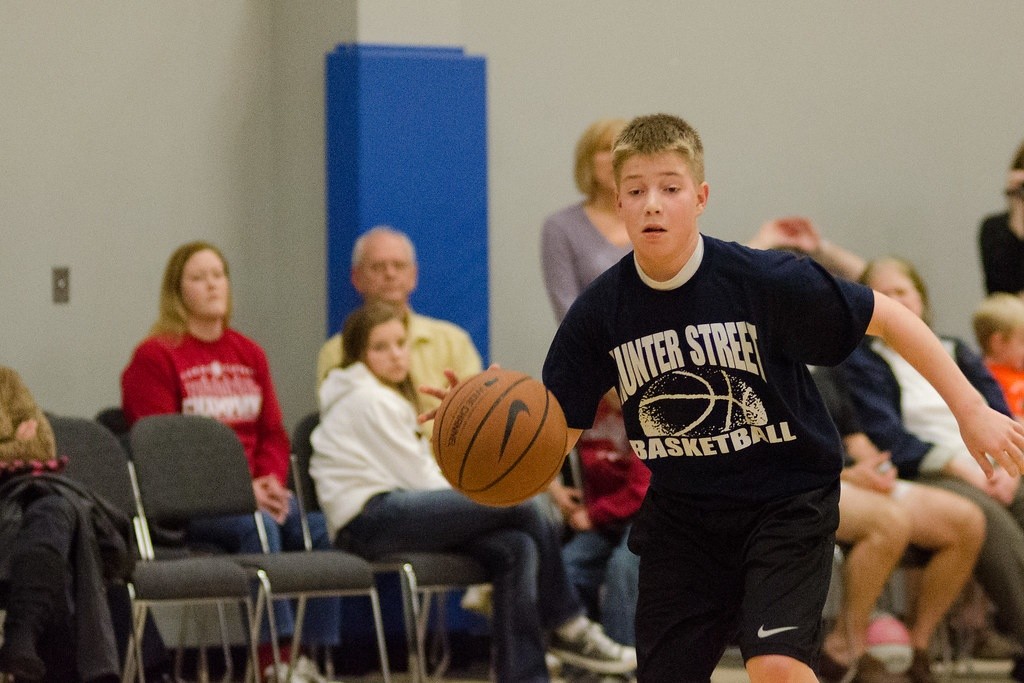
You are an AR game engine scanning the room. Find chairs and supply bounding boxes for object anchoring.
[37,407,505,683]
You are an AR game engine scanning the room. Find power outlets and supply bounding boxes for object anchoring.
[52,267,70,304]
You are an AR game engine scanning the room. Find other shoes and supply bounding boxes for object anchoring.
[907,647,935,683]
[817,644,890,683]
[265,657,326,683]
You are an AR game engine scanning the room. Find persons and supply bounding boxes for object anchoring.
[414,113,1024,682]
[745,219,1024,682]
[976,143,1024,296]
[541,117,655,325]
[0,365,121,683]
[548,381,650,682]
[119,240,328,683]
[308,223,639,683]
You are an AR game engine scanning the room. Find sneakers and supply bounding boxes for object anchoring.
[548,619,638,675]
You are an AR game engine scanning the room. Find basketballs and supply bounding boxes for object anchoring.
[432,368,569,508]
